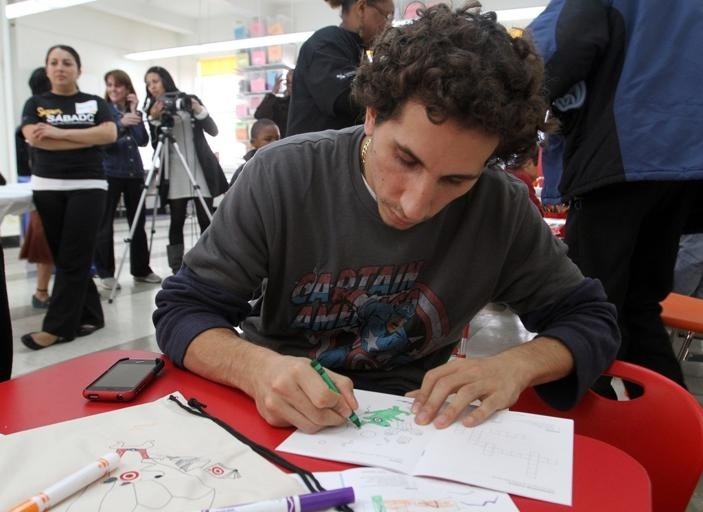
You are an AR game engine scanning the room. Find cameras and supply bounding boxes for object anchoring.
[155,93,192,111]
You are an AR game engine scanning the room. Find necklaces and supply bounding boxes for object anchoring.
[360,136,372,163]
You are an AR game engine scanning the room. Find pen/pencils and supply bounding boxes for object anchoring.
[200,487,354,512]
[9,451,119,512]
[310,359,360,426]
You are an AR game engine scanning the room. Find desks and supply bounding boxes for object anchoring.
[0,347,651,512]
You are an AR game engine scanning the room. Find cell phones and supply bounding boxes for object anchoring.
[81,357,165,402]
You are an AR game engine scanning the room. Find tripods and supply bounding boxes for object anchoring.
[108,127,213,303]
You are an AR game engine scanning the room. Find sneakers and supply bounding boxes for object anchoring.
[102,272,161,290]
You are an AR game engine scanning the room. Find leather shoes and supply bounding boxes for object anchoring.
[22,325,98,350]
[33,295,52,308]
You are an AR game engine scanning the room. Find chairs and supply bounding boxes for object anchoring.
[510,360,703,512]
[658,291,703,362]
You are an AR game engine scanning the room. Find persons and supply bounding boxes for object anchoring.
[286,0,396,138]
[93,68,162,290]
[150,0,624,437]
[229,117,281,186]
[254,69,296,139]
[521,1,703,401]
[18,43,126,351]
[143,66,229,275]
[18,66,55,310]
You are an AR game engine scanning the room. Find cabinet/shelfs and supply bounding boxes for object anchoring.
[236,40,296,155]
